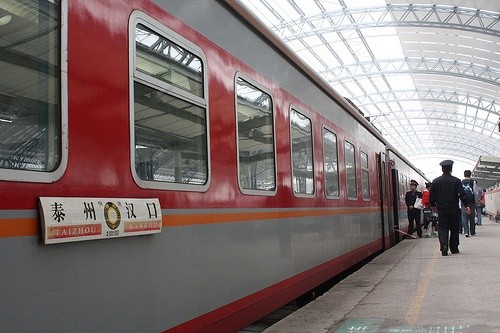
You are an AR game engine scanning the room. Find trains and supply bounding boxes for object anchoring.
[0,0,434,333]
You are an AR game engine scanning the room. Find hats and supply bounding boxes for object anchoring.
[410,180,419,185]
[439,160,454,167]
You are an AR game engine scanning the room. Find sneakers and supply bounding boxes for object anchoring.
[465,234,468,237]
[431,233,437,235]
[471,233,476,235]
[423,234,432,238]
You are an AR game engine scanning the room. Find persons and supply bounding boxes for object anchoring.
[430,160,471,256]
[405,180,423,238]
[422,182,432,236]
[462,170,476,237]
[481,189,486,215]
[430,203,438,235]
[475,180,482,226]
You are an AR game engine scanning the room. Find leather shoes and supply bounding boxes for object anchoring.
[418,235,422,238]
[442,250,448,256]
[452,250,459,254]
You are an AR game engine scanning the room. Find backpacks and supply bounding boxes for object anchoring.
[462,179,474,201]
